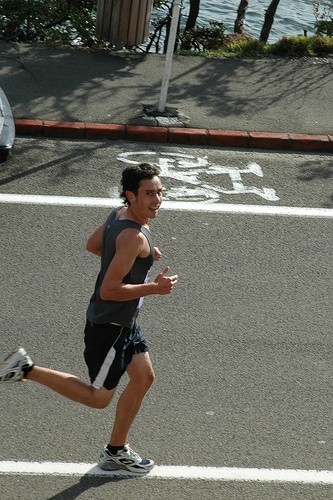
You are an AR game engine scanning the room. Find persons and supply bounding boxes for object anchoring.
[0,162,179,472]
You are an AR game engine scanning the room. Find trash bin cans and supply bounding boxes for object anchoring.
[87,0,158,48]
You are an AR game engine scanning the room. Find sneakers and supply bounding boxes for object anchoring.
[97,443,154,473]
[0,346,34,385]
